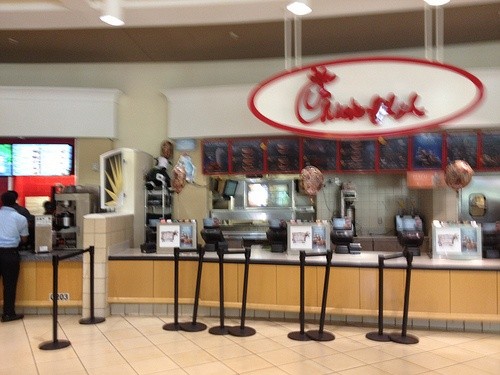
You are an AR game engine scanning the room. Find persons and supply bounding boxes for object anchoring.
[0,194,29,321]
[44,201,51,214]
[8,190,32,226]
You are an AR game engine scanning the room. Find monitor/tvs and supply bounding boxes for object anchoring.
[223,180,238,198]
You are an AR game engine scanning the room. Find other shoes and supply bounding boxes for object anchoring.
[2,313,24,322]
